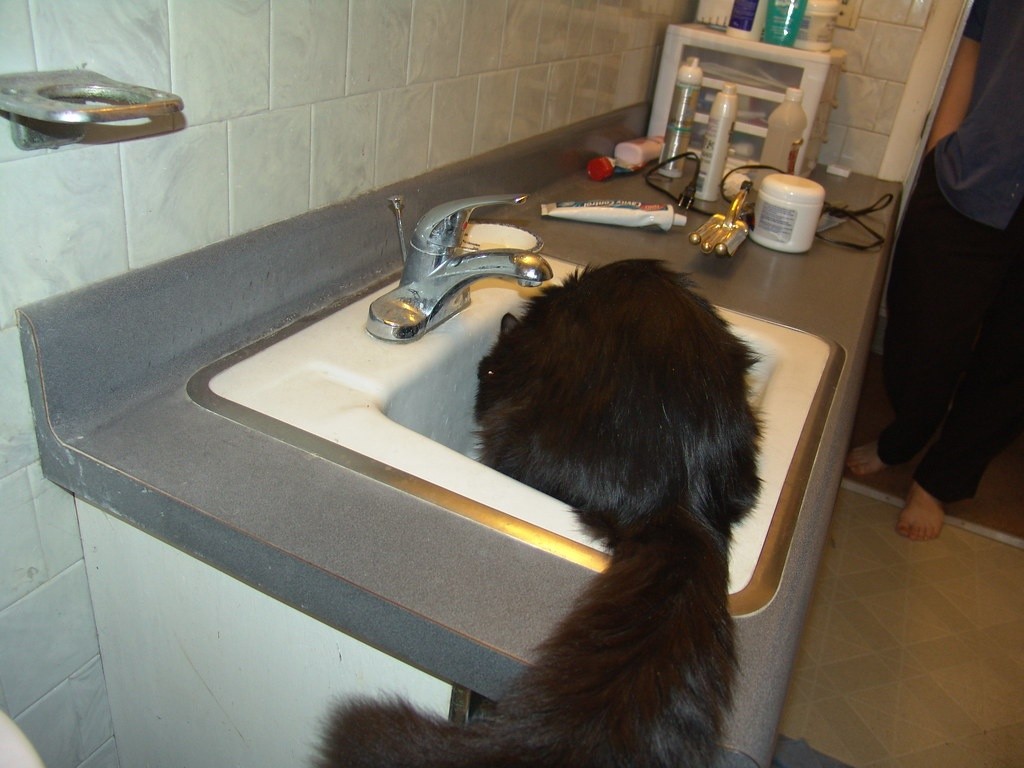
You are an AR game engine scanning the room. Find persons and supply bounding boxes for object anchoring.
[847,0,1024,543]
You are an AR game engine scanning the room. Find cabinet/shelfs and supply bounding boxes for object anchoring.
[645,20,847,178]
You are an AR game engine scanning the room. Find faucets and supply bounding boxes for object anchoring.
[364,193,554,343]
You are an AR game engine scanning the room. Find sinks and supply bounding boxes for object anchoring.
[182,253,846,622]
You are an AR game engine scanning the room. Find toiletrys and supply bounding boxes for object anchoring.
[539,197,688,233]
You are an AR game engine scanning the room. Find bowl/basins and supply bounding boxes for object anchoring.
[460,217,544,253]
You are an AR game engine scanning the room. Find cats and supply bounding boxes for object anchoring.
[308,259,768,768]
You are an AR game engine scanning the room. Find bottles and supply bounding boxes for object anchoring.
[614,135,664,167]
[657,56,702,179]
[726,0,768,41]
[764,0,808,48]
[752,87,808,191]
[692,82,738,202]
